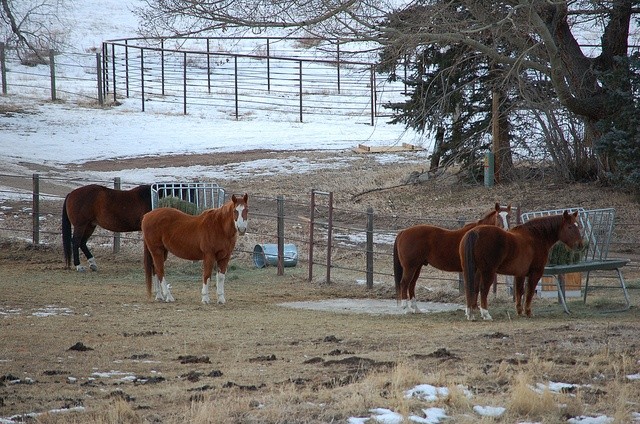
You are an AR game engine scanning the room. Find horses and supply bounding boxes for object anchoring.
[459,209,584,321]
[61,182,200,272]
[393,202,512,315]
[141,192,249,304]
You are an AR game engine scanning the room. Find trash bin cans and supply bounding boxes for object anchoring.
[253,243,297,269]
